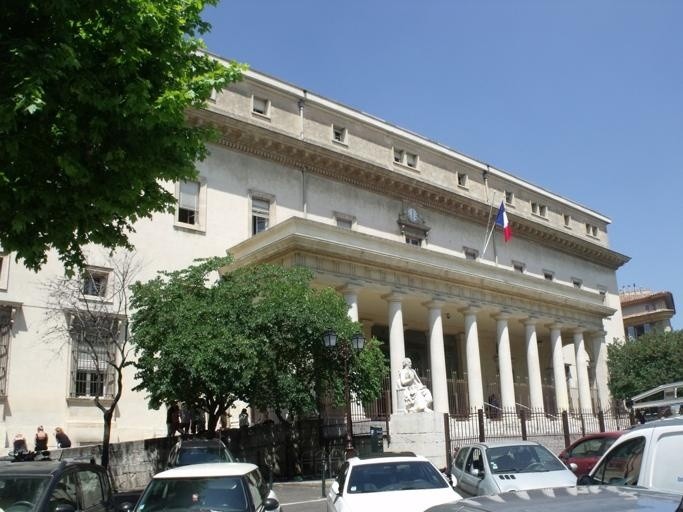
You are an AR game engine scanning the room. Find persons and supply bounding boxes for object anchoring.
[165,397,209,437]
[33,425,48,451]
[54,426,72,449]
[12,432,29,455]
[633,408,645,425]
[255,405,269,425]
[220,410,229,431]
[399,356,433,413]
[239,409,250,430]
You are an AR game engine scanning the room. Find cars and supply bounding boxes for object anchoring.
[559,431,625,479]
[326,451,464,512]
[451,441,579,497]
[164,438,241,471]
[0,456,117,512]
[423,484,683,512]
[120,462,281,512]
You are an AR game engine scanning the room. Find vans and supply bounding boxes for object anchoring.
[575,414,682,495]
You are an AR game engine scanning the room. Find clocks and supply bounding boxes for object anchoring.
[406,207,419,223]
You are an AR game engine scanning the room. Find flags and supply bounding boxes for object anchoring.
[494,201,513,242]
